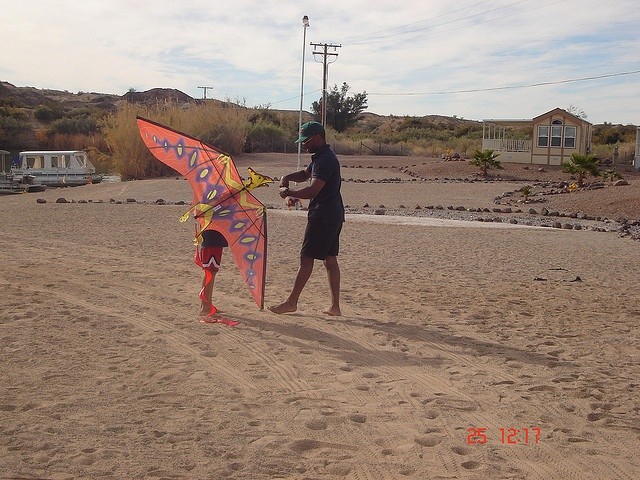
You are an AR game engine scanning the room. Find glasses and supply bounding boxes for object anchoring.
[302,137,314,145]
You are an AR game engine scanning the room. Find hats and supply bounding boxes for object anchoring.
[296,121,324,144]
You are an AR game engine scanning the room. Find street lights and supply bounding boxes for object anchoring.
[297,15,310,164]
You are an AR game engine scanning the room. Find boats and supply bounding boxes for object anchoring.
[10,150,102,187]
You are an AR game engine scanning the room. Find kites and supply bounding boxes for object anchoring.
[135,115,275,314]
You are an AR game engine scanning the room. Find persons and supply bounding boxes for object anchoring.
[195,157,234,316]
[266,122,346,314]
